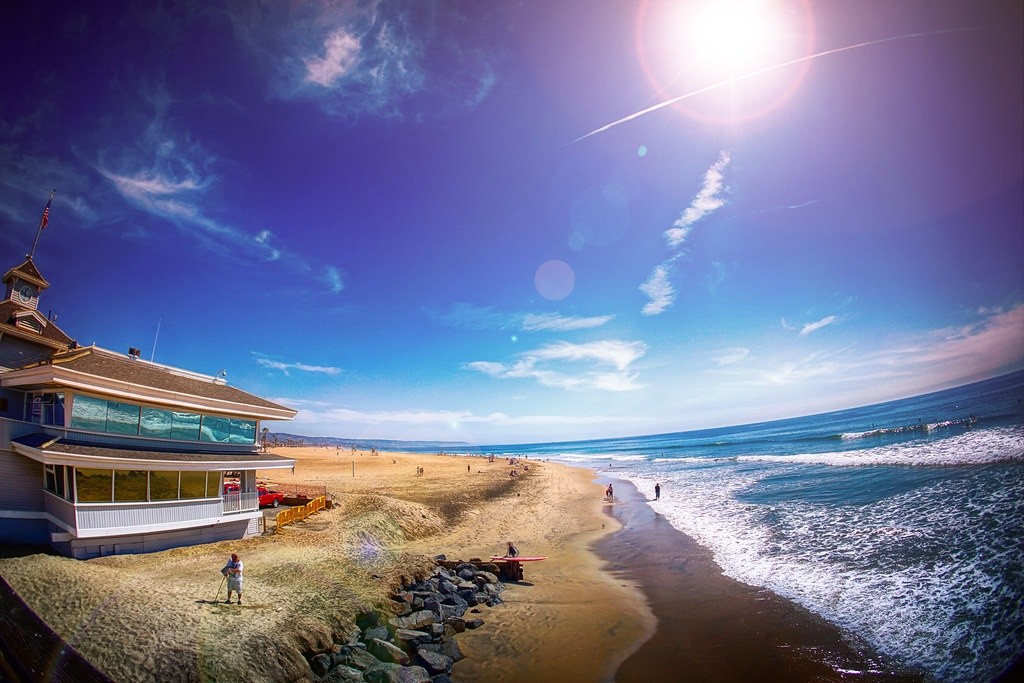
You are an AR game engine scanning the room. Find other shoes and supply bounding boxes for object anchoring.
[238,601,241,605]
[225,600,232,604]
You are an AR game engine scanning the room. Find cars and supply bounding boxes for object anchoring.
[225,484,284,508]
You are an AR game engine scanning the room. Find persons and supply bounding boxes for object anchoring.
[508,457,528,476]
[416,466,424,475]
[655,483,660,499]
[491,457,493,461]
[606,489,609,497]
[225,554,243,605]
[609,484,613,500]
[468,464,471,472]
[506,542,520,557]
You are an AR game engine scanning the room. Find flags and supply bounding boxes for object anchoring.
[42,200,51,230]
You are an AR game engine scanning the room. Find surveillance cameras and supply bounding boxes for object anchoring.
[222,372,226,376]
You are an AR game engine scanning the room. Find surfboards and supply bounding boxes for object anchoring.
[491,556,548,561]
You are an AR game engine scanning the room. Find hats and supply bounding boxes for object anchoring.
[232,554,238,560]
